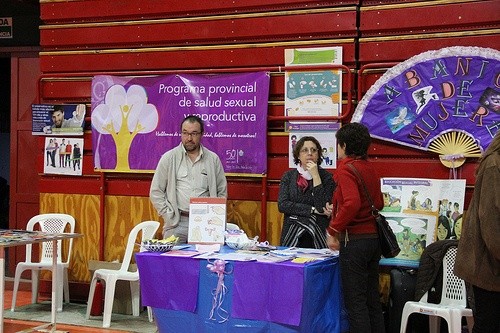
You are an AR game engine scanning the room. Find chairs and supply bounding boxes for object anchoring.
[397,240,473,333]
[85,220,161,327]
[11,213,76,312]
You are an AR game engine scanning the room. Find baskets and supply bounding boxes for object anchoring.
[142,244,174,252]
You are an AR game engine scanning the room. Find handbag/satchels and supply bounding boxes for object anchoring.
[372,208,401,259]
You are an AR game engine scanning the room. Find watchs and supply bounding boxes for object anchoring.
[312,207,316,213]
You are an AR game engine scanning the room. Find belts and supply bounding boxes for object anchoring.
[178,208,189,217]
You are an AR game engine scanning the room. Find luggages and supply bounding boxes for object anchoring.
[389,267,419,333]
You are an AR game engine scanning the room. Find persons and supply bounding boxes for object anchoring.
[50,104,85,128]
[278,136,336,249]
[46,139,58,167]
[326,122,384,333]
[73,143,81,171]
[453,134,500,333]
[149,116,227,244]
[65,140,72,168]
[59,139,66,168]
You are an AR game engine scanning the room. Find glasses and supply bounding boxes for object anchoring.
[299,147,319,153]
[181,130,202,135]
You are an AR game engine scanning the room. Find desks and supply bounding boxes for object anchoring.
[133,244,341,333]
[0,229,79,333]
